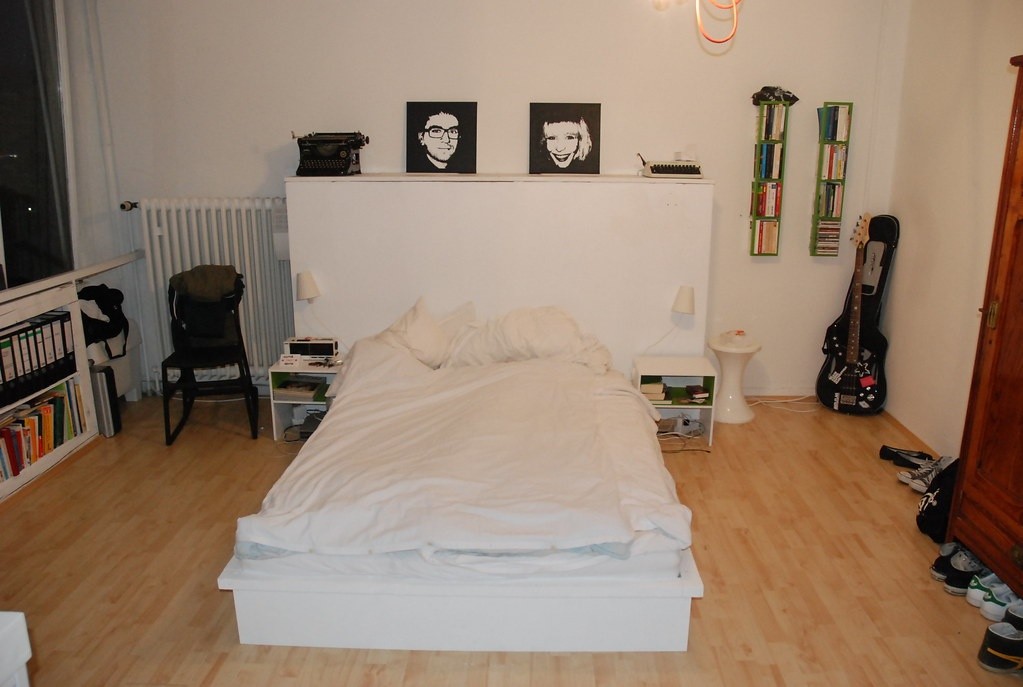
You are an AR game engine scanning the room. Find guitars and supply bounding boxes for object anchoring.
[814,210,890,418]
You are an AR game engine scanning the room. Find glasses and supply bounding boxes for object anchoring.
[423,127,463,140]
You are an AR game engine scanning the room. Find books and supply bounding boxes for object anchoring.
[751,182,781,218]
[817,221,840,256]
[820,184,842,217]
[641,376,672,405]
[273,376,326,402]
[823,144,846,179]
[756,104,786,143]
[0,378,86,482]
[749,221,778,255]
[754,144,783,179]
[686,385,710,398]
[817,106,848,142]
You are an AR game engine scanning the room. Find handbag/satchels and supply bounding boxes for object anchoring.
[915,458,959,545]
[75,284,131,358]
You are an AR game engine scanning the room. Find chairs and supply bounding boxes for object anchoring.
[160,264,259,446]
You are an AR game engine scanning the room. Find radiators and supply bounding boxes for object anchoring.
[138,198,296,387]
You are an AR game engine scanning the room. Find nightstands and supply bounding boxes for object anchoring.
[268,357,344,441]
[630,355,719,447]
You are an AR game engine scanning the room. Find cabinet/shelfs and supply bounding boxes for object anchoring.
[0,281,100,501]
[944,55,1023,601]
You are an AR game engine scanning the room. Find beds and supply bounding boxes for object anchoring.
[216,338,705,653]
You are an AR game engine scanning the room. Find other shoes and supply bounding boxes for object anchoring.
[879,444,1023,674]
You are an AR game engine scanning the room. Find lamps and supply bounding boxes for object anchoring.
[296,270,321,304]
[670,285,696,315]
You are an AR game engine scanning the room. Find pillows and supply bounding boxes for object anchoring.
[376,295,615,371]
